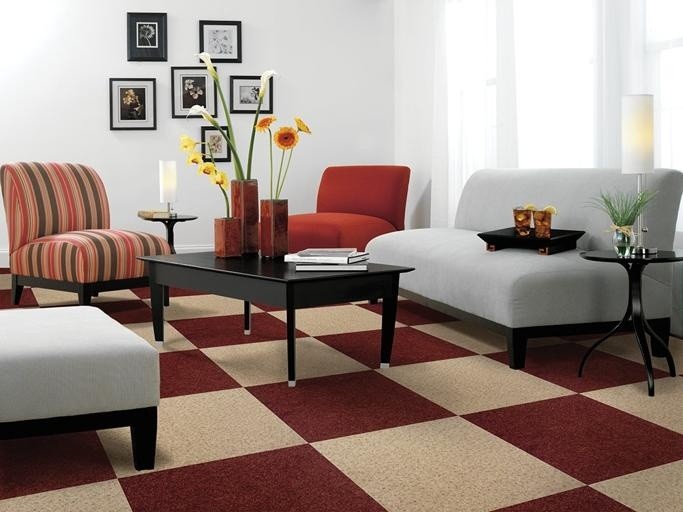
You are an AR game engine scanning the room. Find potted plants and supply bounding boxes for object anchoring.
[584,186,657,257]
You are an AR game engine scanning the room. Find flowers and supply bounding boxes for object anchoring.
[178,137,229,218]
[187,52,278,179]
[256,115,312,201]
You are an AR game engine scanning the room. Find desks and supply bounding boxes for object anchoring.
[576,248,683,396]
[137,212,198,254]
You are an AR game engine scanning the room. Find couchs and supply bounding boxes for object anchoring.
[0,304,160,471]
[273,163,410,250]
[365,167,683,371]
[0,160,172,311]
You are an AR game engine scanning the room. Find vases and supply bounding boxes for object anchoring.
[259,198,288,259]
[228,180,258,255]
[213,217,240,258]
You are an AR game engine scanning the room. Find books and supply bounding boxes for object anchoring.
[282,247,369,272]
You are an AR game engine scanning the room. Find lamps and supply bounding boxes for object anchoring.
[620,91,658,255]
[157,161,179,213]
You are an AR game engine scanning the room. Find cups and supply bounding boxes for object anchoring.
[531,210,551,239]
[512,207,531,236]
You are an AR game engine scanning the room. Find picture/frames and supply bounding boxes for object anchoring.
[126,13,169,63]
[170,66,218,118]
[108,78,156,129]
[228,75,273,115]
[199,126,229,162]
[198,19,243,64]
